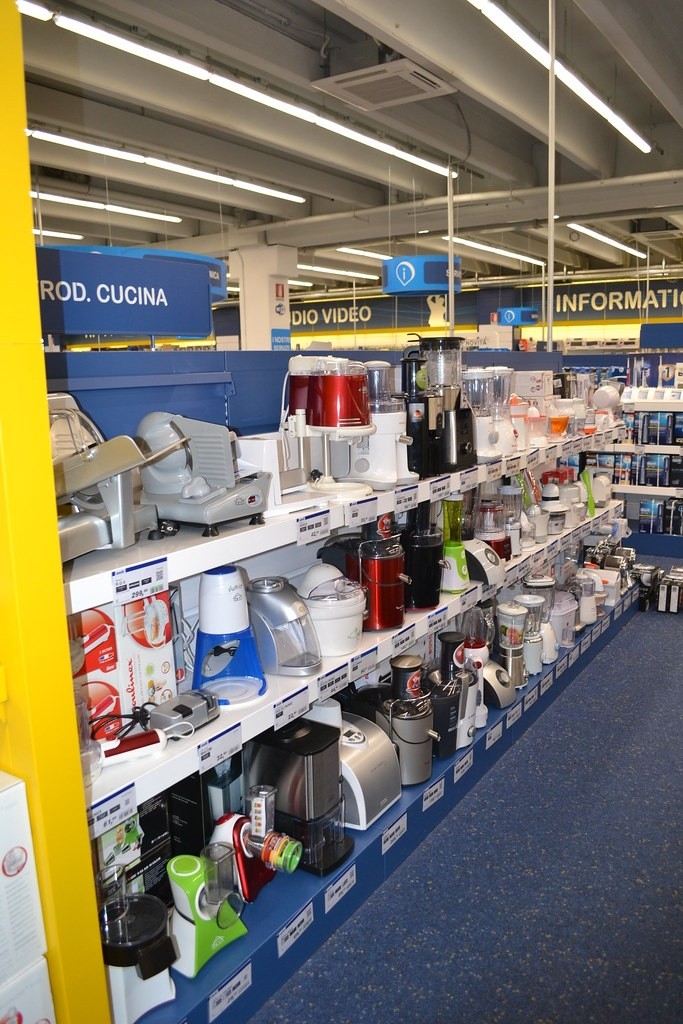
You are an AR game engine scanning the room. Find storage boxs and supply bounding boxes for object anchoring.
[512,371,554,416]
[583,534,621,569]
[89,788,175,909]
[553,367,625,399]
[0,772,58,1024]
[630,564,683,614]
[172,752,246,857]
[556,411,683,489]
[639,496,683,535]
[68,591,177,745]
[354,551,570,689]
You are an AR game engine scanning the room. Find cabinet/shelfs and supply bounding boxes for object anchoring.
[44,350,645,1024]
[565,353,683,559]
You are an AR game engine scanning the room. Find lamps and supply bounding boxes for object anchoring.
[466,0,654,153]
[15,0,460,179]
[26,127,307,204]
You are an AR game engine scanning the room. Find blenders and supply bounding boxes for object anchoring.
[47,335,638,1024]
